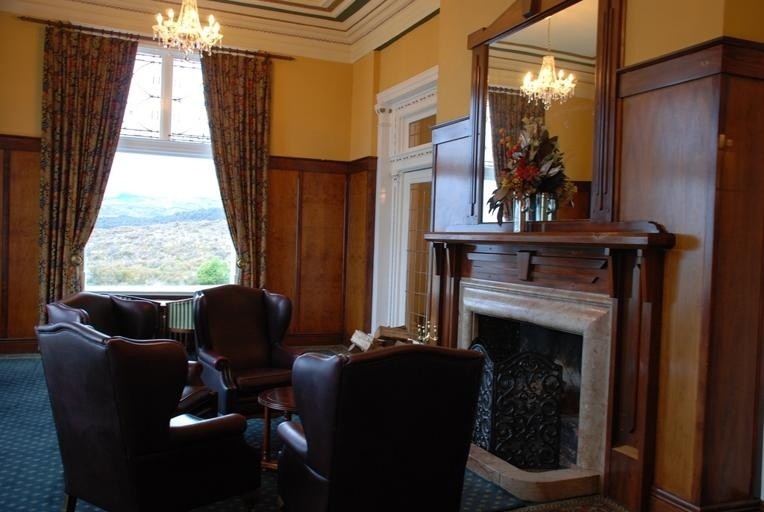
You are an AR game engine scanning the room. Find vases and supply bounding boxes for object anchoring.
[539,194,553,221]
[514,199,525,232]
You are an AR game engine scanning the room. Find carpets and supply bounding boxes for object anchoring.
[519,493,625,511]
[294,344,352,356]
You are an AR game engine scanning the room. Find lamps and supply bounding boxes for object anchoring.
[151,0,224,55]
[520,14,576,103]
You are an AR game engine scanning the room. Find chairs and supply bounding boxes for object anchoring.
[268,343,486,511]
[193,283,300,416]
[32,322,247,511]
[43,289,219,416]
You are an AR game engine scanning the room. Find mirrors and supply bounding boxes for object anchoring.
[467,0,628,223]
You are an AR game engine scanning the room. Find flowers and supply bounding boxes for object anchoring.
[527,120,576,203]
[489,117,542,198]
